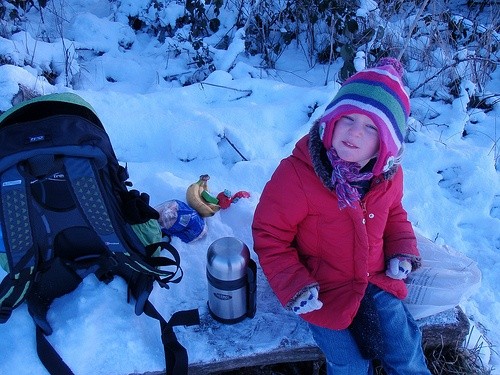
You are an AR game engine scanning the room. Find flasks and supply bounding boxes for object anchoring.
[205,237,257,326]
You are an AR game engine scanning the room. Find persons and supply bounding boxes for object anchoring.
[252,58,431,375]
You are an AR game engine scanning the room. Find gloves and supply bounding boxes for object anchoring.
[287,288,323,314]
[382,256,412,281]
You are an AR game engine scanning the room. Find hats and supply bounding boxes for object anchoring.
[318,57,412,182]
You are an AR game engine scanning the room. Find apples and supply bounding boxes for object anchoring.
[217,192,232,208]
[232,191,251,199]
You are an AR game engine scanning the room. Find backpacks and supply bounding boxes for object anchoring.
[0,92,183,327]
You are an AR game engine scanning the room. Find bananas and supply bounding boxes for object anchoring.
[185,175,222,216]
